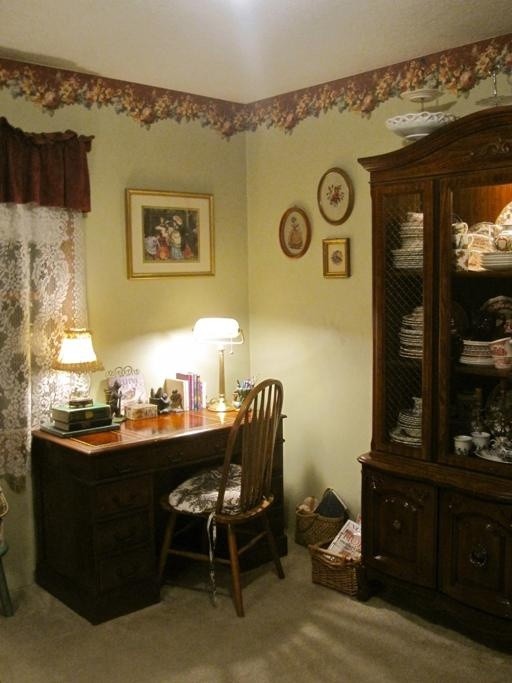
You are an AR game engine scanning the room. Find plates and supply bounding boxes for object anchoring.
[398,306,425,359]
[389,408,423,450]
[390,209,424,269]
[452,299,471,343]
[400,88,445,102]
[385,111,467,140]
[458,340,492,366]
[469,201,512,272]
[480,249,512,270]
[471,448,512,464]
[479,295,512,335]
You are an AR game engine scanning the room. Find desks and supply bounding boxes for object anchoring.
[32,398,286,626]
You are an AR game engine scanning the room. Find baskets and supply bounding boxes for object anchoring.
[296,509,344,547]
[308,538,360,596]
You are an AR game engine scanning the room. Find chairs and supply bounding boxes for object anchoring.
[157,378,284,616]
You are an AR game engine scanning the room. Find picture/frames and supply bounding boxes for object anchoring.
[323,238,349,279]
[124,188,215,281]
[318,168,355,226]
[280,206,313,258]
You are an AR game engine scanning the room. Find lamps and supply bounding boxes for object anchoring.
[191,315,244,413]
[55,326,104,400]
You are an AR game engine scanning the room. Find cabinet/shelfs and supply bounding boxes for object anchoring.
[356,105,512,653]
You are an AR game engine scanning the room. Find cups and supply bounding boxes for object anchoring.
[492,355,512,371]
[454,434,473,456]
[472,431,492,450]
[490,336,512,355]
[452,221,474,273]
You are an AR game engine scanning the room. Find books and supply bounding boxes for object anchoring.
[40,421,121,440]
[323,520,361,561]
[311,487,350,520]
[167,372,206,412]
[52,418,112,431]
[51,402,112,423]
